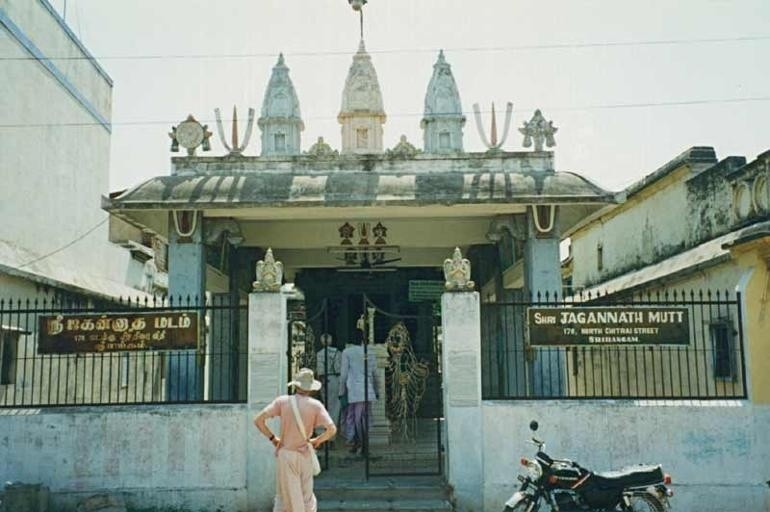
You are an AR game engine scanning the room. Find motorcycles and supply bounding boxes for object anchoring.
[495,417,673,511]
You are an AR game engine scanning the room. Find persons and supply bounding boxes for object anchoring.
[335,327,383,458]
[249,366,339,512]
[314,332,350,453]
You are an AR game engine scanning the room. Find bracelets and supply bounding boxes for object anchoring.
[268,434,275,441]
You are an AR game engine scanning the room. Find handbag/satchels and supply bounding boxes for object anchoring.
[305,443,321,476]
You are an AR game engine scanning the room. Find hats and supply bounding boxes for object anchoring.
[288,368,322,391]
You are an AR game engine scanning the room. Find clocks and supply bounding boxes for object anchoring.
[175,121,205,150]
